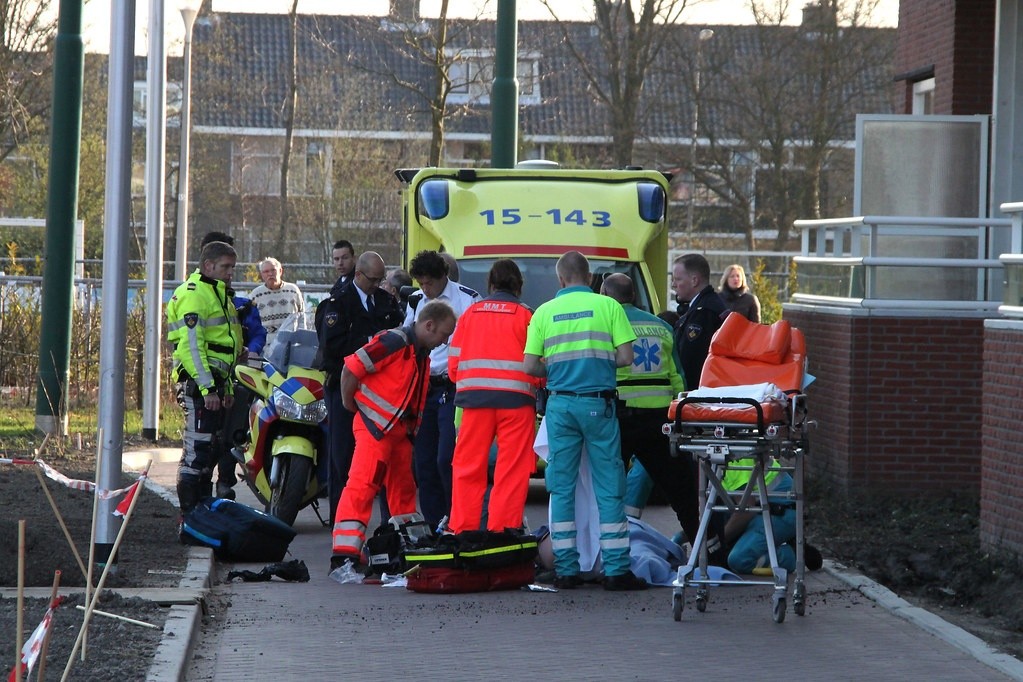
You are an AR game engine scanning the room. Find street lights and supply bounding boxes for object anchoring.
[176,7,199,282]
[686,30,713,249]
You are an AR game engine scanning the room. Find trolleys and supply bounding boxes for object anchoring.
[662,389,818,623]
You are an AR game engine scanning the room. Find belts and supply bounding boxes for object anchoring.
[548,391,605,397]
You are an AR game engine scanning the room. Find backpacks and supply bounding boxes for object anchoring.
[177,497,298,562]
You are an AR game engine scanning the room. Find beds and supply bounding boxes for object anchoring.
[664,320,808,622]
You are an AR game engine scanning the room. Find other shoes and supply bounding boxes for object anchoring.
[216,482,235,499]
[604,570,647,591]
[329,557,360,576]
[786,533,822,570]
[554,575,577,588]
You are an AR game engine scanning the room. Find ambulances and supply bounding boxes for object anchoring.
[393,159,674,477]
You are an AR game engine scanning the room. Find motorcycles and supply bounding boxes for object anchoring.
[230,312,330,527]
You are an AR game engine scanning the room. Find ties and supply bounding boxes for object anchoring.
[366,295,375,313]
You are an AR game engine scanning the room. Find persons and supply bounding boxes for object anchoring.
[599,254,746,572]
[314,239,548,535]
[327,299,456,581]
[522,250,646,590]
[446,258,547,534]
[712,452,824,576]
[248,256,307,361]
[716,264,761,324]
[193,231,268,501]
[165,242,244,521]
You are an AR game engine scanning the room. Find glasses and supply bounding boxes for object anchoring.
[361,271,386,282]
